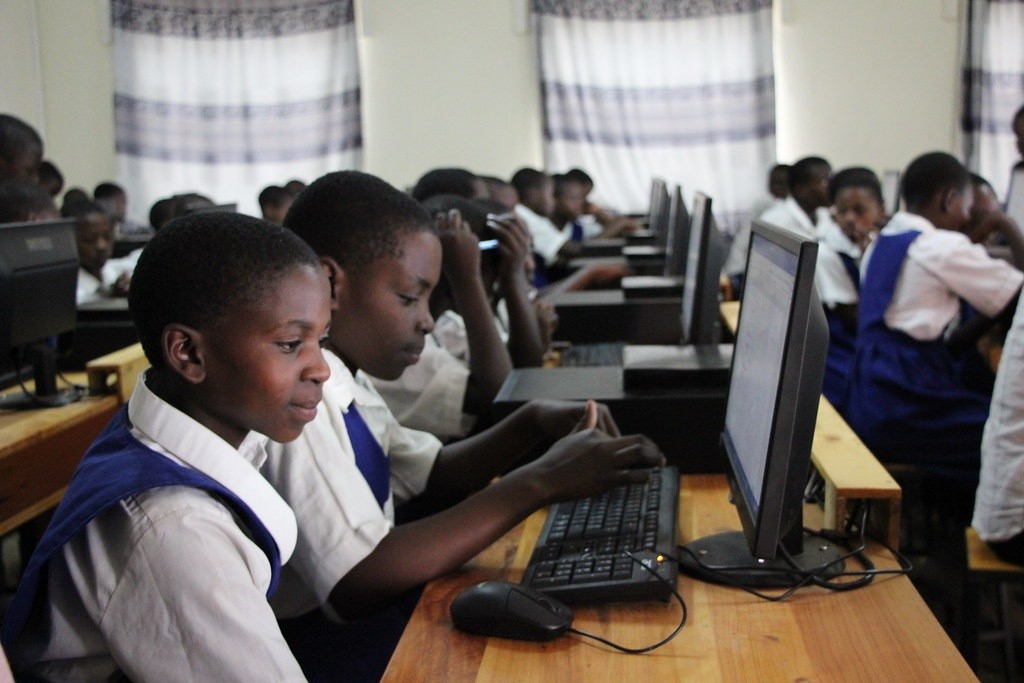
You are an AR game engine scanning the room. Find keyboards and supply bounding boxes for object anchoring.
[561,341,627,370]
[521,466,680,605]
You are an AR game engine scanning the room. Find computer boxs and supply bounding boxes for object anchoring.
[496,236,734,477]
[113,234,154,259]
[64,296,142,375]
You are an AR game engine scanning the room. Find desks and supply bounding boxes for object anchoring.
[0,214,1014,683]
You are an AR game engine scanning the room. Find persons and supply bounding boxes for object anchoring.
[0,210,332,683]
[0,103,1024,683]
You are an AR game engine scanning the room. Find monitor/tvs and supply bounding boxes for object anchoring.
[644,177,847,588]
[187,203,237,215]
[882,171,900,219]
[0,217,81,410]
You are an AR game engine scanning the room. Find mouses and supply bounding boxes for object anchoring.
[449,579,576,642]
